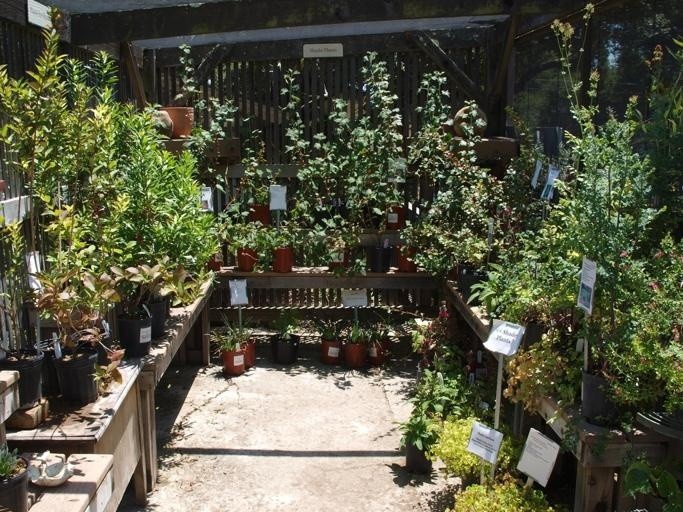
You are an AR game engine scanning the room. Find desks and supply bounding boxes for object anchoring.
[443,278,655,510]
[202,265,440,368]
[12,275,216,512]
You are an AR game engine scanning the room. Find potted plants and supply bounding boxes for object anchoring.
[207,49,424,273]
[0,438,32,512]
[452,227,492,308]
[398,418,441,475]
[202,304,411,380]
[0,24,203,410]
[579,100,666,430]
